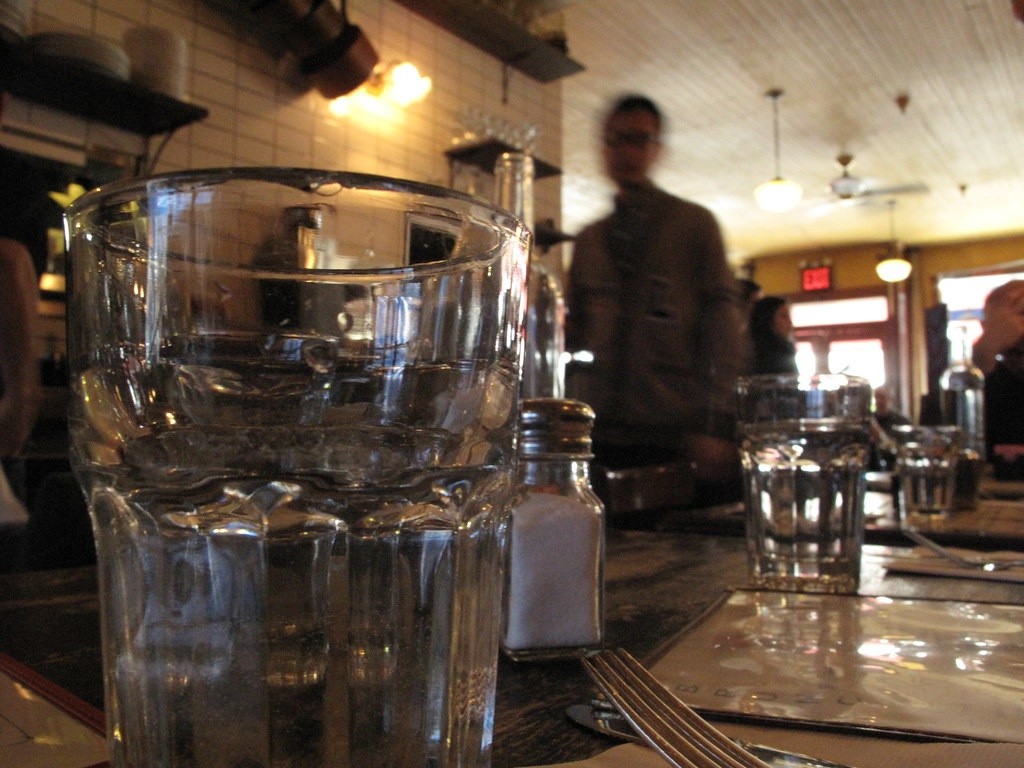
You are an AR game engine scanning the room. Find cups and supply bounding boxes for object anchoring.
[730,370,874,593]
[890,425,964,526]
[62,165,534,768]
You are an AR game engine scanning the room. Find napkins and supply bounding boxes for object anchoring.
[536,720,1024,768]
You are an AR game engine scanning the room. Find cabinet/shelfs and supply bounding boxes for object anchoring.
[445,138,577,254]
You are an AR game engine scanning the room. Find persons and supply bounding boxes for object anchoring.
[973,279,1024,479]
[565,95,798,483]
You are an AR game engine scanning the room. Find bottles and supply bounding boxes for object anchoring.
[491,152,566,397]
[938,324,986,495]
[495,397,607,663]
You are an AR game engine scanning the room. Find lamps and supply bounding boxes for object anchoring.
[753,89,804,214]
[874,206,913,283]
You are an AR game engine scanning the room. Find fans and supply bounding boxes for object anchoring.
[804,154,931,215]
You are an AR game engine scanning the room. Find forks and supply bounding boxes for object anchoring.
[580,647,771,768]
[894,527,1024,567]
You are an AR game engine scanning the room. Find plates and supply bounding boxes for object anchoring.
[0,0,188,105]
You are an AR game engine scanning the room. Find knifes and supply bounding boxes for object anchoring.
[565,704,855,768]
[865,551,939,561]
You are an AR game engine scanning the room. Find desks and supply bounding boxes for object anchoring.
[0,529,1024,768]
[662,487,1024,553]
[864,472,1024,501]
